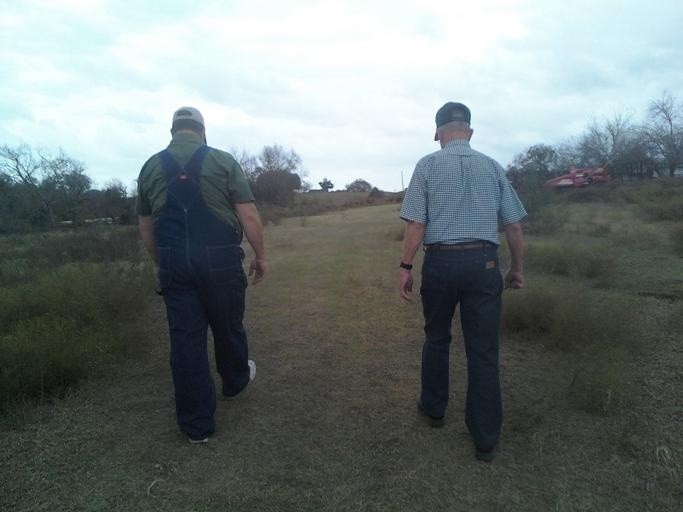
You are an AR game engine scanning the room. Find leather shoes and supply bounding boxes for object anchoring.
[417,393,446,427]
[474,448,497,462]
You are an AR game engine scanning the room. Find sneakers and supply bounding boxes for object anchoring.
[188,437,208,444]
[224,360,256,399]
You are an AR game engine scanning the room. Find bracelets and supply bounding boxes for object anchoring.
[400,261,414,270]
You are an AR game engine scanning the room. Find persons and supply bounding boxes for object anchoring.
[135,106,269,445]
[399,101,528,463]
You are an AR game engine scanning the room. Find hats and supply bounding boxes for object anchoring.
[172,106,205,128]
[434,101,471,141]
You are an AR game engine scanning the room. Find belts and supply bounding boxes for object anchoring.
[424,243,497,250]
